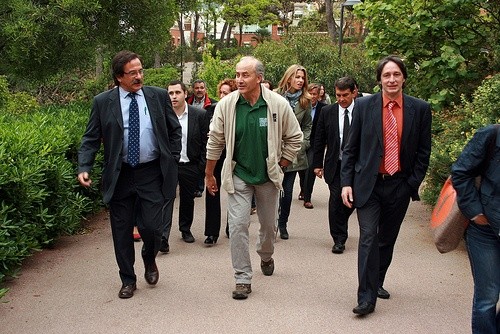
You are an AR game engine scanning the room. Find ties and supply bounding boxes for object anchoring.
[341,109,349,151]
[127,92,140,168]
[384,102,399,177]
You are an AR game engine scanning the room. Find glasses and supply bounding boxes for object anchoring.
[124,71,144,77]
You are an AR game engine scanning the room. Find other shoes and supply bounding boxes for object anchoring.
[225,218,229,238]
[250,207,257,215]
[133,233,141,241]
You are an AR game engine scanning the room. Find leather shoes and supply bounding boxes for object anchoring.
[353,302,375,315]
[143,258,159,285]
[118,283,136,298]
[194,191,202,197]
[299,192,304,200]
[278,228,288,239]
[332,244,345,253]
[205,235,218,244]
[376,286,390,299]
[182,231,194,242]
[304,201,313,208]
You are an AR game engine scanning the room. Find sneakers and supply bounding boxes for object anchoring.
[261,257,274,276]
[232,284,251,299]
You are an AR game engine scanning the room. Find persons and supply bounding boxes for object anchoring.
[133,64,373,255]
[339,56,431,316]
[450,124,500,334]
[77,51,182,298]
[205,56,304,299]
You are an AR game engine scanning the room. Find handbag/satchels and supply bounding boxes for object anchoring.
[430,174,481,255]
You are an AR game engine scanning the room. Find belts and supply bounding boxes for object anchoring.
[377,174,402,181]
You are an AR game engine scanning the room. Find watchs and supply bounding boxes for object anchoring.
[279,164,288,171]
